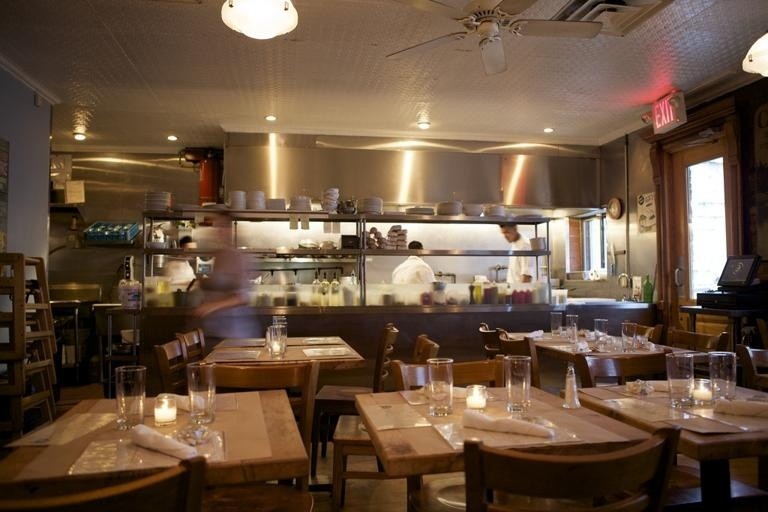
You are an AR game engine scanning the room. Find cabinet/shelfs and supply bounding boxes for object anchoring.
[143,208,553,358]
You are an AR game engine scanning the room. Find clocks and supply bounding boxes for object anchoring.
[607,198,622,219]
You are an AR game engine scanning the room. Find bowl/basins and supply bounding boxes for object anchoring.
[145,241,168,249]
[321,188,358,215]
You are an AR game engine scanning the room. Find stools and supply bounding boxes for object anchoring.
[0,252,57,441]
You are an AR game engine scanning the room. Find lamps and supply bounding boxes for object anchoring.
[742,32,767,77]
[220,0,299,41]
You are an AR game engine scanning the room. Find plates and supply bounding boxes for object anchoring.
[358,197,543,219]
[143,190,310,213]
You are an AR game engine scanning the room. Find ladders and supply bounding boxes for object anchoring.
[0,253,58,438]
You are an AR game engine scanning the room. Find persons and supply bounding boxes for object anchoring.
[162,236,197,283]
[500,223,534,284]
[392,241,435,284]
[186,211,262,354]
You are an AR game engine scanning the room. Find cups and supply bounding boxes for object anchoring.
[550,311,655,353]
[665,351,737,408]
[426,355,532,417]
[339,276,357,306]
[551,289,568,305]
[265,315,288,359]
[115,362,217,430]
[529,237,547,251]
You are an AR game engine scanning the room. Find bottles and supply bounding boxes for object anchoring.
[330,272,340,306]
[311,270,321,306]
[643,274,653,303]
[421,279,546,305]
[66,215,81,249]
[562,365,582,410]
[320,271,330,306]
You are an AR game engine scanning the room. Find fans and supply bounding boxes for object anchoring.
[387,0,603,77]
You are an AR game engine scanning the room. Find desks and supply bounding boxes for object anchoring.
[1,389,308,510]
[197,337,365,375]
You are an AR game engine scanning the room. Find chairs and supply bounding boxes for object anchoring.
[151,337,200,395]
[175,327,209,375]
[202,362,318,510]
[309,306,767,512]
[0,456,209,512]
